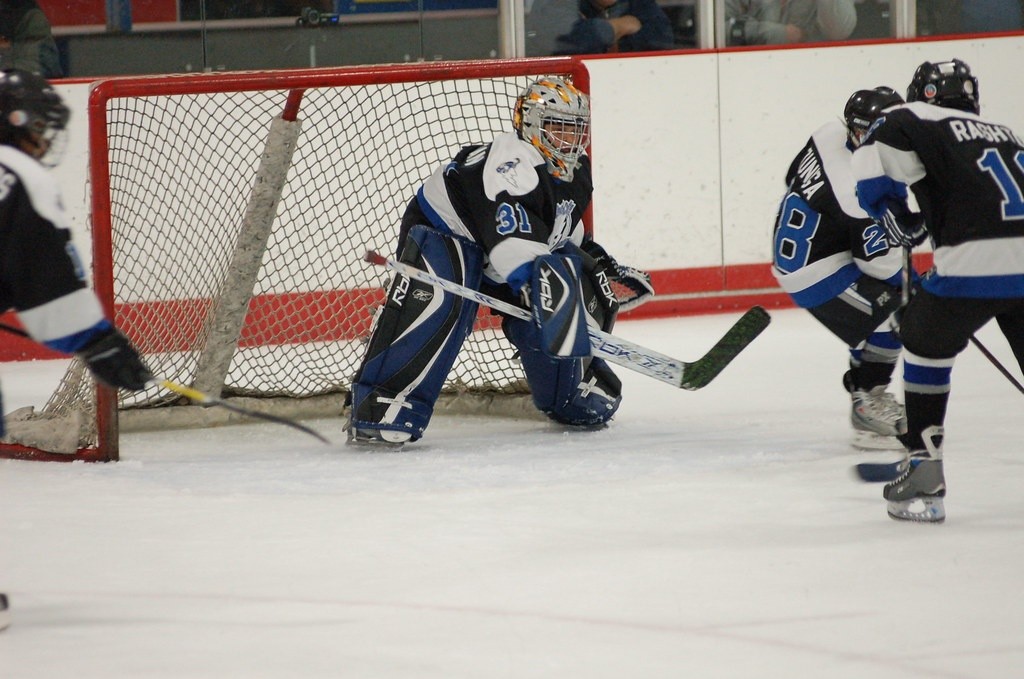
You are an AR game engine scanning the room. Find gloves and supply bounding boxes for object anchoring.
[76,323,154,391]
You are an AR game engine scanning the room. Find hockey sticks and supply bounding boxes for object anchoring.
[1,321,334,446]
[361,248,774,392]
[969,331,1024,394]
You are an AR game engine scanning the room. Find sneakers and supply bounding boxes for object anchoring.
[842,377,908,450]
[882,425,948,523]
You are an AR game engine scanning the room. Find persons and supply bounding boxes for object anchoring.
[0,0,64,77]
[341,76,654,446]
[522,0,1024,522]
[0,69,154,633]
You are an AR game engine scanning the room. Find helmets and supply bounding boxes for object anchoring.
[844,85,906,134]
[0,68,70,146]
[511,75,591,182]
[906,58,981,116]
